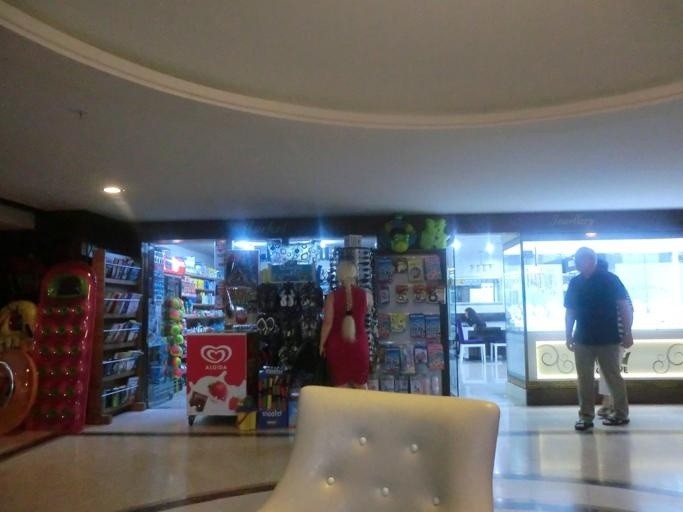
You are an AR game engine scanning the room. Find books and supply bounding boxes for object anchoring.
[103,256,143,408]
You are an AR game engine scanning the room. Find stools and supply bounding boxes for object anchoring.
[490,342,506,363]
[459,344,487,365]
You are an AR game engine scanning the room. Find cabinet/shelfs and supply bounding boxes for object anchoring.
[148,271,223,405]
[77,236,144,426]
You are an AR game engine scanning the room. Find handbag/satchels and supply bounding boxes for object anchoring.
[294,348,329,387]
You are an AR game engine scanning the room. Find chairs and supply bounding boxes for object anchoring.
[259,384,501,512]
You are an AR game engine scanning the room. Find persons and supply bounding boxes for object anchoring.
[317,260,368,391]
[463,306,485,328]
[596,261,633,419]
[562,246,633,431]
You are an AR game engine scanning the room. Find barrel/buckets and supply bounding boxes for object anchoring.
[235,405,258,431]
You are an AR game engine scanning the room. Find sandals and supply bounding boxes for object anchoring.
[575,420,594,431]
[598,405,630,426]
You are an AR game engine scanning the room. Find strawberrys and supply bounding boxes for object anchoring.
[208,382,226,400]
[229,397,238,410]
